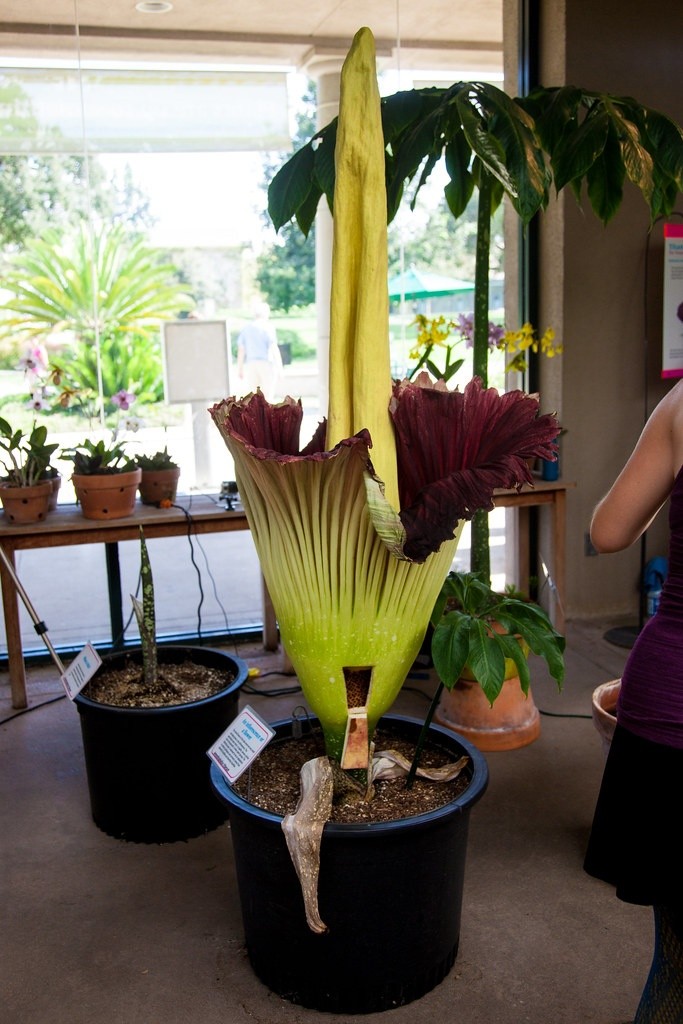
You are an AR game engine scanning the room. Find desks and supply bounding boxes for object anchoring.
[0,483,569,707]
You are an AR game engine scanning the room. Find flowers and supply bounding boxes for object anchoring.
[406,311,564,395]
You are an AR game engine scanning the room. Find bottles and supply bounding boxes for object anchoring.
[648,578,664,618]
[542,437,560,481]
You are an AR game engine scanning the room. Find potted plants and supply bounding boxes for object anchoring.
[429,571,565,750]
[210,26,489,1015]
[0,415,180,525]
[66,524,249,843]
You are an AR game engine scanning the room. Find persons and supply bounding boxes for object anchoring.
[582,378,683,1024]
